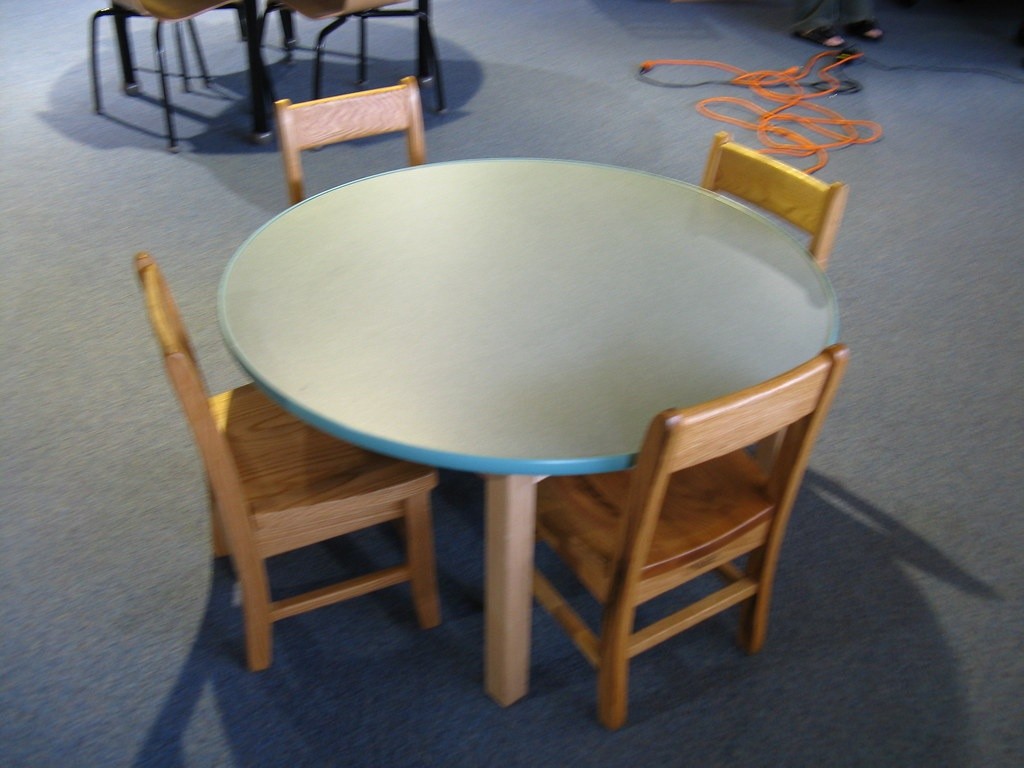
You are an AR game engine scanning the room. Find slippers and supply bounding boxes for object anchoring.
[797,26,845,47]
[847,20,883,41]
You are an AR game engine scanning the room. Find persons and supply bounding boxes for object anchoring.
[788,0,886,48]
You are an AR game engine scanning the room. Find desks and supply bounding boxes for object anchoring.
[214,158,840,707]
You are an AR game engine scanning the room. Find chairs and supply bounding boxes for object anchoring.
[700,132,849,274]
[273,76,427,205]
[86,0,448,155]
[133,252,443,672]
[534,344,849,732]
